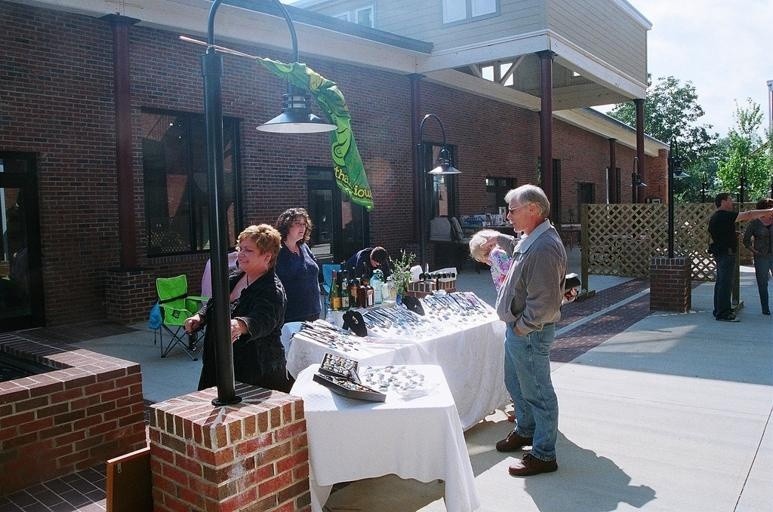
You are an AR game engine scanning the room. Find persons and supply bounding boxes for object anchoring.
[709,194,773,321]
[469,230,579,425]
[479,184,567,474]
[184,224,288,389]
[348,246,390,281]
[743,198,772,311]
[272,206,321,320]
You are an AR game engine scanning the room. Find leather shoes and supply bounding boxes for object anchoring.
[507,452,558,477]
[494,429,536,453]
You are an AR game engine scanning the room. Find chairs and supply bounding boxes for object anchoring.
[153,274,210,361]
[321,264,342,318]
[450,217,482,273]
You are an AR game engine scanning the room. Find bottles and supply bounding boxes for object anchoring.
[408,272,456,292]
[329,269,395,309]
[481,206,508,226]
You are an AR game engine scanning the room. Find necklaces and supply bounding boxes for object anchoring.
[246,274,262,286]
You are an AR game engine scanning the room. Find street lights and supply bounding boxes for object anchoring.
[199,0,338,410]
[699,172,712,226]
[629,156,648,237]
[735,169,756,231]
[668,135,690,258]
[415,112,462,284]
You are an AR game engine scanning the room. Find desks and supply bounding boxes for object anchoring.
[280,291,509,434]
[288,365,479,511]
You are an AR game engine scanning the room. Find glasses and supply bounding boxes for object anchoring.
[507,202,534,214]
[294,222,307,228]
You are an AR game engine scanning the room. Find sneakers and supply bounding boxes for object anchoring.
[761,307,771,315]
[712,308,740,323]
